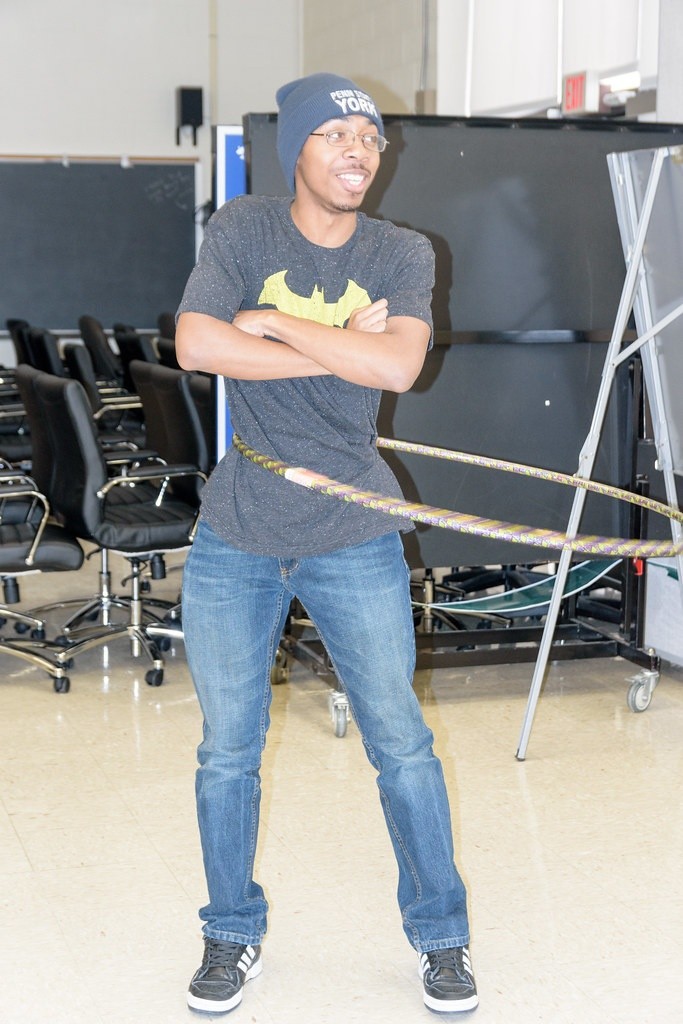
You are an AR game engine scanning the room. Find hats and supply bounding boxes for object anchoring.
[275,71,384,196]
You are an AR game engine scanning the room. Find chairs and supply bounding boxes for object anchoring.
[0,312,210,694]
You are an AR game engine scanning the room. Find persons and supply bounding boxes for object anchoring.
[174,73,481,1016]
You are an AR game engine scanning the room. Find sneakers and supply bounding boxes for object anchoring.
[187,937,262,1012]
[418,945,478,1016]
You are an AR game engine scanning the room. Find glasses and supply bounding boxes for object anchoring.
[310,128,390,153]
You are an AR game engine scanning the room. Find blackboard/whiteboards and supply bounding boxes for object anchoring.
[0,154,203,340]
